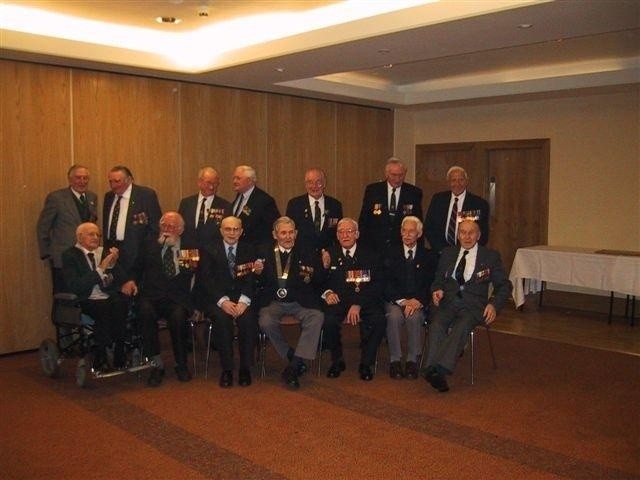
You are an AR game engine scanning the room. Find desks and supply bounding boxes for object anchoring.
[506,239,640,333]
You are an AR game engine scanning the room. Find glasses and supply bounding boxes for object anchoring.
[225,227,240,232]
[339,229,356,235]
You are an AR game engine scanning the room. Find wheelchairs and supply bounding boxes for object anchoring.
[37,262,165,391]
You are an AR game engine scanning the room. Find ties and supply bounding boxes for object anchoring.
[227,247,236,280]
[447,198,458,246]
[196,198,207,236]
[163,245,176,281]
[109,196,123,242]
[87,253,104,293]
[234,193,244,217]
[283,251,288,257]
[314,201,321,234]
[455,250,469,286]
[346,249,352,261]
[406,250,414,266]
[390,187,396,213]
[80,193,85,202]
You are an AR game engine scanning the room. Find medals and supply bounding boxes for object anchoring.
[274,287,288,298]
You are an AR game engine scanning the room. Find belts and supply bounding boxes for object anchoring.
[87,295,113,304]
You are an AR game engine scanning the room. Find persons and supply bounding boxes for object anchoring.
[35,164,99,360]
[122,210,204,385]
[285,167,343,352]
[384,214,435,379]
[359,156,424,238]
[425,218,512,394]
[254,216,333,390]
[193,215,264,389]
[423,167,491,357]
[176,164,231,351]
[314,218,386,381]
[61,222,133,375]
[102,166,163,282]
[231,164,283,340]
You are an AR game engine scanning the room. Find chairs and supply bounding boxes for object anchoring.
[140,314,497,386]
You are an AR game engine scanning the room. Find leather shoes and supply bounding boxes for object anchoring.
[93,352,111,372]
[281,353,346,389]
[219,370,234,387]
[175,365,191,382]
[113,350,127,371]
[390,360,450,392]
[148,366,165,386]
[358,365,374,380]
[238,368,251,386]
